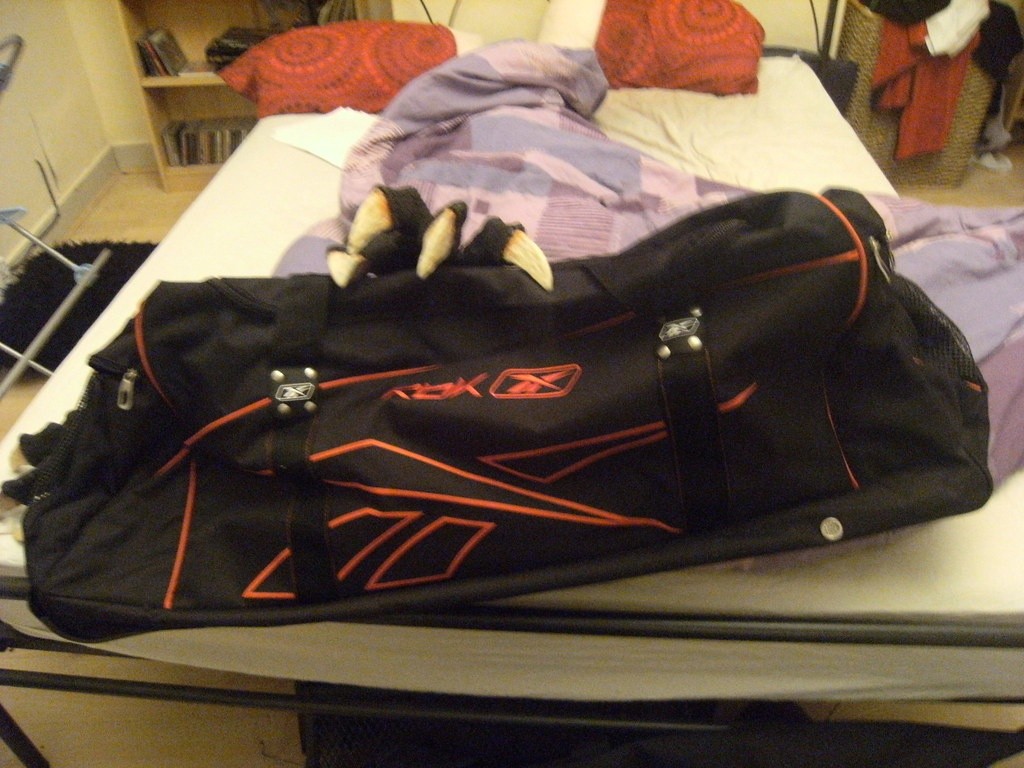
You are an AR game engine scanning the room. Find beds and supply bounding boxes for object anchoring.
[0,1,1023,768]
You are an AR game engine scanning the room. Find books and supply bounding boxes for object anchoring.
[136,26,187,78]
[204,27,268,71]
[161,116,255,167]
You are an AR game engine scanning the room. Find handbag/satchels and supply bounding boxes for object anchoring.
[24,186,993,646]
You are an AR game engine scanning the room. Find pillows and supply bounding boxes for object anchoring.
[539,0,766,99]
[215,19,479,121]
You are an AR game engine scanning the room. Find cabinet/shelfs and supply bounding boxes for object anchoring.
[114,0,367,192]
